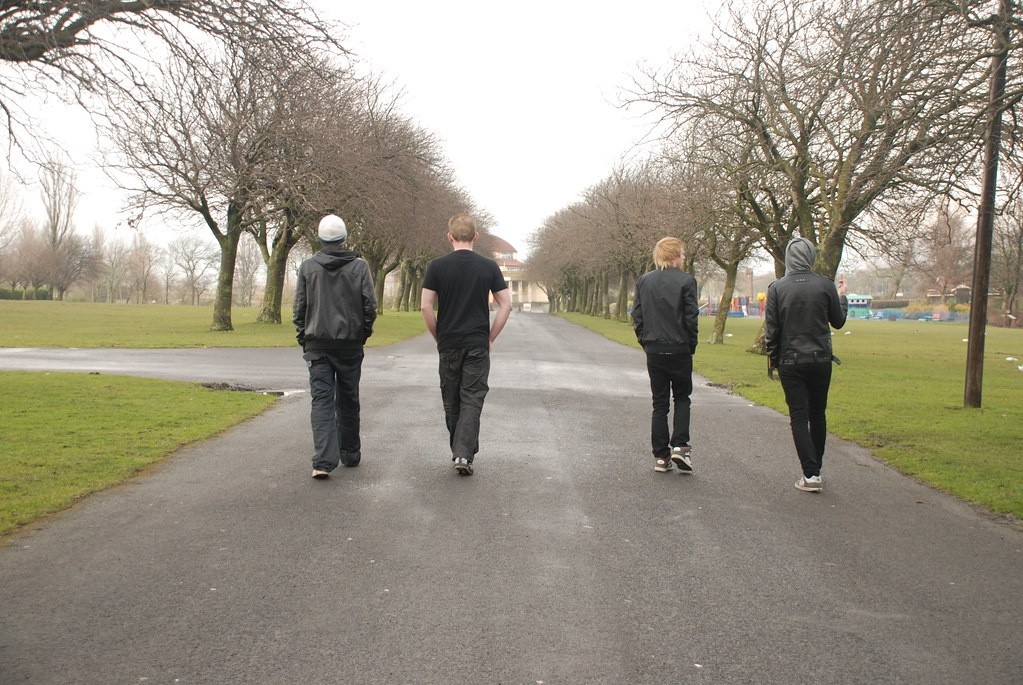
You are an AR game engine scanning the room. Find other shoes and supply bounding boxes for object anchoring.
[312,468,330,477]
[454,457,473,477]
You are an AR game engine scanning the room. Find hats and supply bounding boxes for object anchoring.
[317,214,347,246]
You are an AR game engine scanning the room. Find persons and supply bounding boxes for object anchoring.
[765,238,847,493]
[421,214,512,477]
[630,238,699,474]
[293,215,378,479]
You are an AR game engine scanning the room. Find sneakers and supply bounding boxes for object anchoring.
[672,443,694,471]
[794,475,822,492]
[655,454,674,472]
